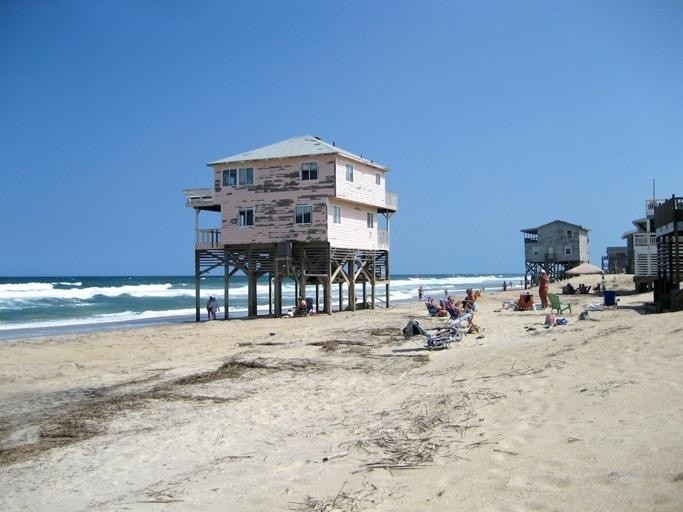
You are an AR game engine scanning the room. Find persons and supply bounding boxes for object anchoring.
[206,298,220,320]
[281,308,294,318]
[205,296,213,321]
[415,269,607,320]
[292,296,307,316]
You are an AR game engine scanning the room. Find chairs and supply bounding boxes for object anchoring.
[645,294,671,313]
[444,300,472,336]
[562,283,600,294]
[293,298,313,317]
[546,293,572,316]
[425,313,472,332]
[423,300,439,319]
[513,294,533,311]
